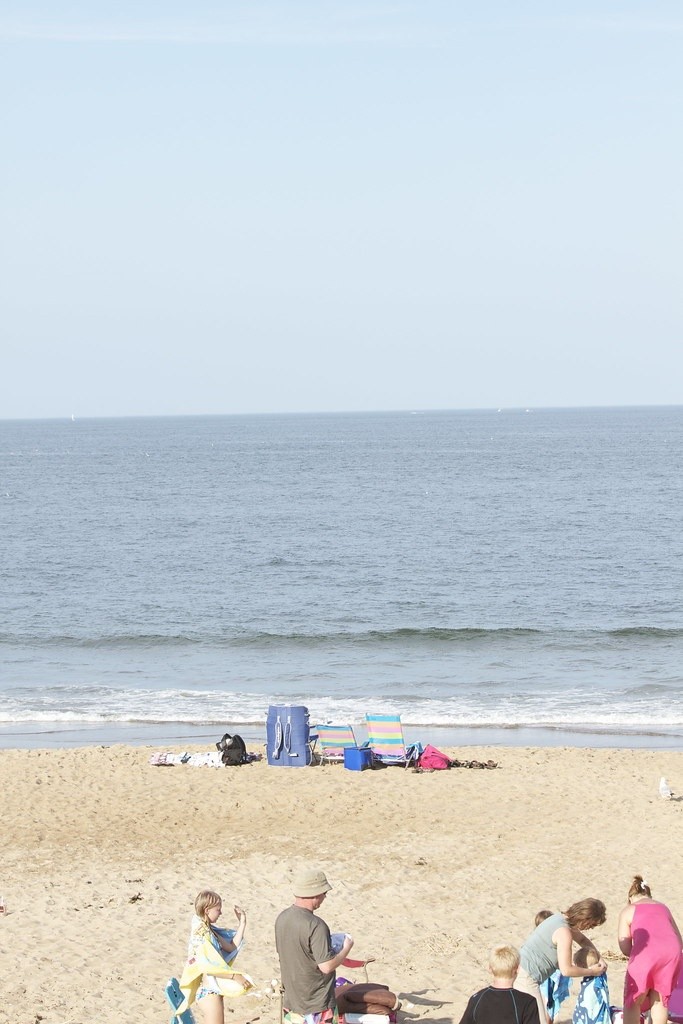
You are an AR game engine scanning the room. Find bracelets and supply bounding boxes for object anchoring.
[232,974,235,979]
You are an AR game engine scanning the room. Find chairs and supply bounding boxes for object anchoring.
[315,724,369,766]
[365,713,421,770]
[278,958,376,1024]
[309,726,319,766]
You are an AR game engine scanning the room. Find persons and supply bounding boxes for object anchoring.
[617,875,683,1024]
[535,910,574,1024]
[173,890,258,1024]
[458,945,540,1024]
[512,897,608,1024]
[275,870,355,1024]
[572,946,613,1024]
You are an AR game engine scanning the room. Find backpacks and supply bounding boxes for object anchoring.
[216,733,247,765]
[419,744,451,772]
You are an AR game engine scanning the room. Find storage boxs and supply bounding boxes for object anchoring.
[344,747,371,771]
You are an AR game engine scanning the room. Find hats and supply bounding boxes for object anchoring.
[291,870,333,899]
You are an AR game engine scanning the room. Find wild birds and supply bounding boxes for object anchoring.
[659,776,675,801]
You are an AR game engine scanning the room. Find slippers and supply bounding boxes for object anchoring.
[247,752,263,762]
[454,758,499,769]
